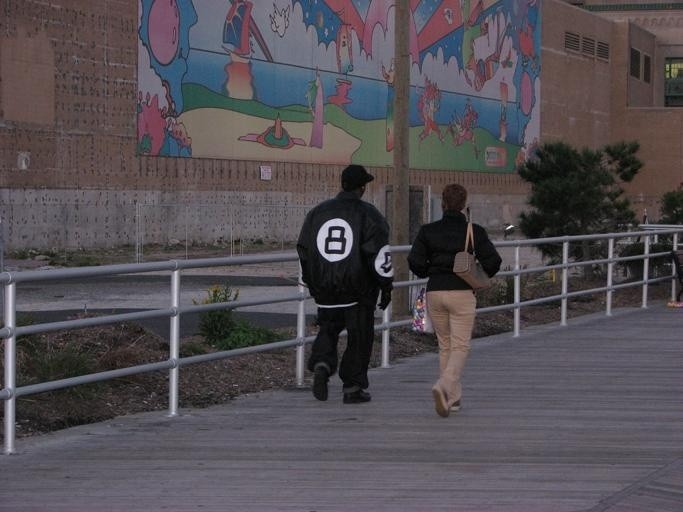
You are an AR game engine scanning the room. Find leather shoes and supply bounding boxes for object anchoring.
[343,387,370,404]
[311,363,330,400]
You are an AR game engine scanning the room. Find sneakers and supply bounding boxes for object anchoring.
[431,383,449,417]
[450,399,463,411]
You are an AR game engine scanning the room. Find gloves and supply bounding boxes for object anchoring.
[378,290,391,311]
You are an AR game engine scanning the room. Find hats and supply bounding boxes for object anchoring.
[342,164,374,188]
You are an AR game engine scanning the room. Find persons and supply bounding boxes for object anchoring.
[407,185,503,418]
[295,165,395,402]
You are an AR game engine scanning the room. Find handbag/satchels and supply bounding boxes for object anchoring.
[411,287,434,336]
[453,221,491,290]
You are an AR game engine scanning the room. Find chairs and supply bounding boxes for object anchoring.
[671,250,682,302]
